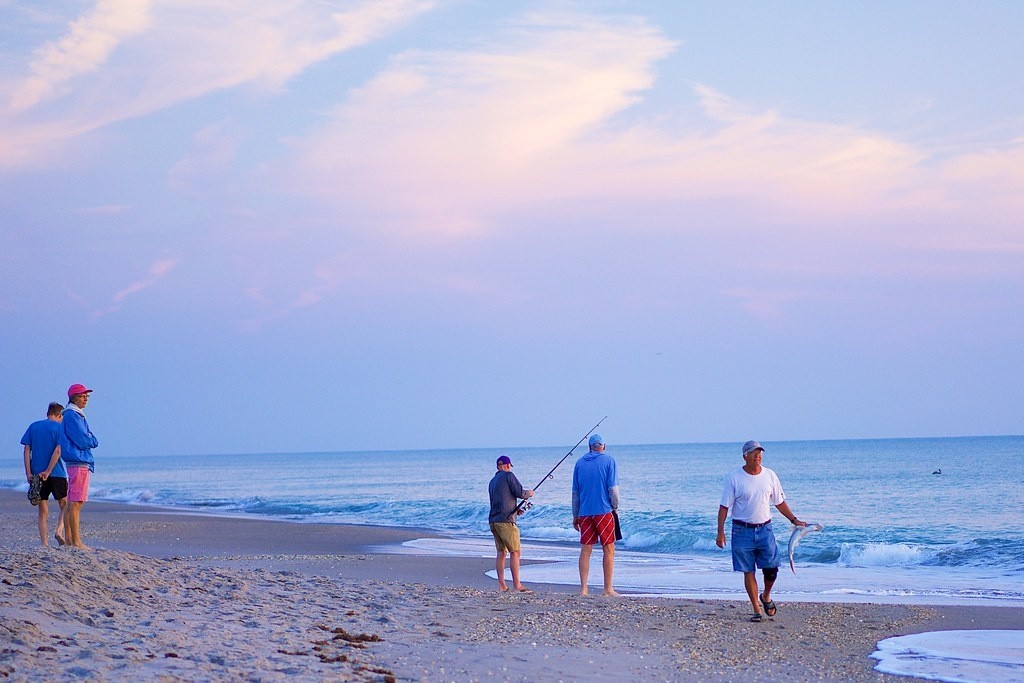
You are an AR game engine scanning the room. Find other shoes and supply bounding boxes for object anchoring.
[27,475,43,506]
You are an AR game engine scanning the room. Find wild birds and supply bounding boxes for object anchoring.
[932,469,941,474]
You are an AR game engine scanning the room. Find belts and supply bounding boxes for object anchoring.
[732,519,771,528]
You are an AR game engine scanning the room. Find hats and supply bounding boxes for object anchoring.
[496,455,514,467]
[743,440,765,454]
[68,384,93,397]
[589,434,605,448]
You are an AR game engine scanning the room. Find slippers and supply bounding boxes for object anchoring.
[513,588,534,594]
[750,614,762,622]
[502,587,513,594]
[760,593,777,616]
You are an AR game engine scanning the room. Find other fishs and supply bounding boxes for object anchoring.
[788,523,823,575]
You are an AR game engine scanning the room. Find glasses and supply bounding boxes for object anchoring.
[76,395,89,398]
[749,452,762,455]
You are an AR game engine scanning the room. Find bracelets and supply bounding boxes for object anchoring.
[612,506,618,509]
[791,517,797,523]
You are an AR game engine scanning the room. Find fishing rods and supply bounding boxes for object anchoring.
[504,415,608,522]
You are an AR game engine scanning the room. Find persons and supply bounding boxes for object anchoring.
[59,383,99,551]
[572,434,623,597]
[20,402,69,547]
[489,456,535,593]
[715,440,808,622]
